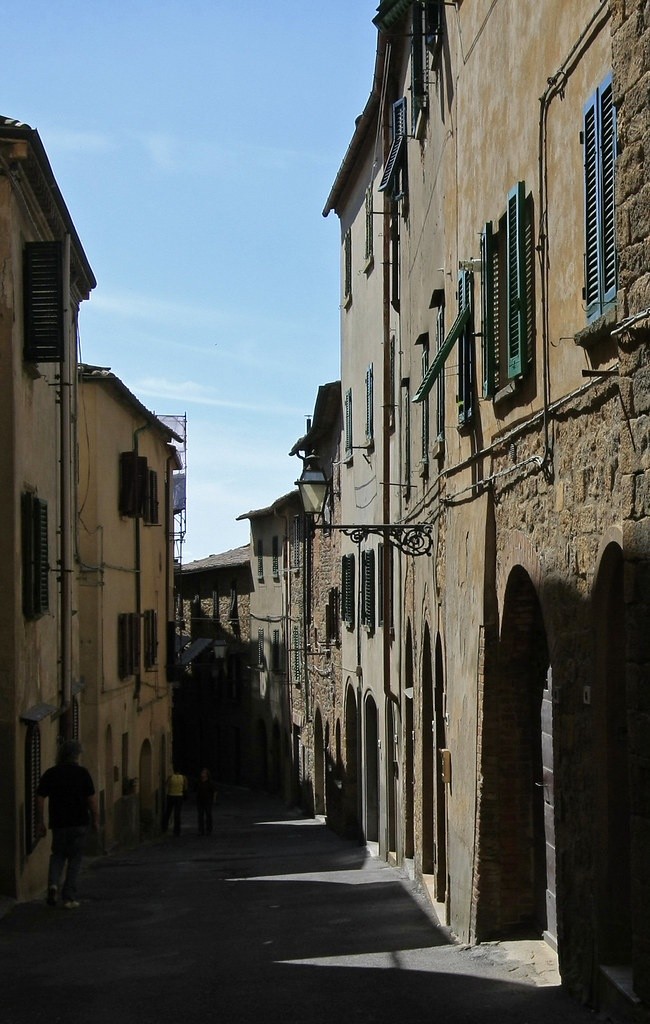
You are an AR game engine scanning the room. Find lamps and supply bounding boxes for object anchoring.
[295,447,435,557]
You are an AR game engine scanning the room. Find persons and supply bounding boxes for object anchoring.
[160,765,187,833]
[35,739,99,908]
[197,769,213,834]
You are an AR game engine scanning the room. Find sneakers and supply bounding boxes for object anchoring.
[63,899,80,909]
[47,884,58,905]
